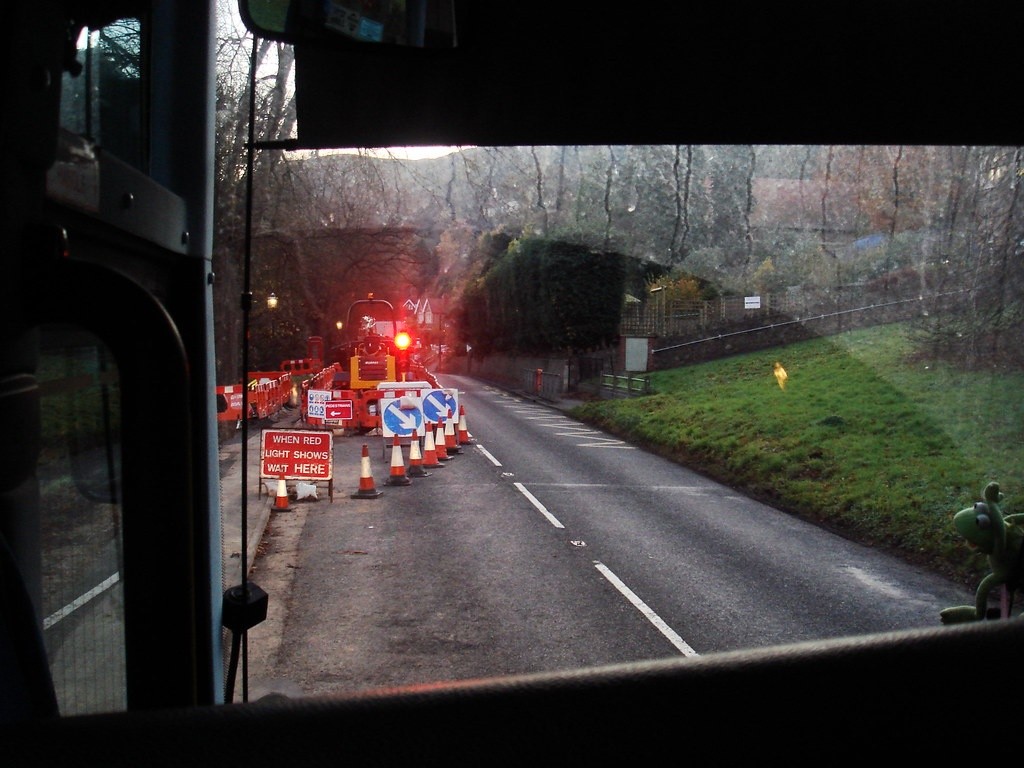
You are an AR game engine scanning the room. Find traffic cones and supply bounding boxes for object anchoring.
[435,417,456,462]
[270,471,297,512]
[349,444,384,499]
[384,433,413,487]
[443,409,461,453]
[420,421,445,468]
[405,428,433,477]
[459,404,471,446]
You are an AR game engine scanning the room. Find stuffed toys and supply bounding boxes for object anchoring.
[940,482,1024,624]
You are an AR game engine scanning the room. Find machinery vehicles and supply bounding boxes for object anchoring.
[330,292,420,389]
